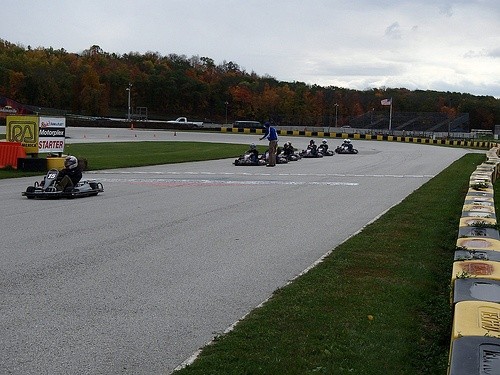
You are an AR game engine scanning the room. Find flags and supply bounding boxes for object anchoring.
[381,98,392,105]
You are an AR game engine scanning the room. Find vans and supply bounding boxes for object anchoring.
[233,121,262,132]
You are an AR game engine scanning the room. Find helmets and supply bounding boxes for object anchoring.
[250,143,256,150]
[64,156,78,169]
[344,139,347,142]
[347,139,351,143]
[310,139,315,144]
[322,139,327,143]
[284,143,289,149]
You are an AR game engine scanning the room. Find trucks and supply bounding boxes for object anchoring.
[176,117,204,127]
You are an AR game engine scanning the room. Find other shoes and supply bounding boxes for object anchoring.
[266,164,274,167]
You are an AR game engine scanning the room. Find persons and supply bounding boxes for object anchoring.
[245,139,353,162]
[259,122,278,167]
[40,156,83,191]
